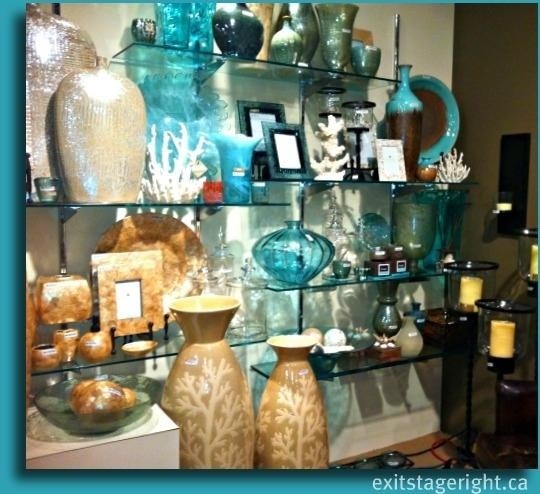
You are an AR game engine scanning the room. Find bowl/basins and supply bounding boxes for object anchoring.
[33,371,162,438]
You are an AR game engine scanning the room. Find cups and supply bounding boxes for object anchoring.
[354,266,369,283]
[34,177,61,204]
[352,45,381,78]
[203,180,224,205]
[333,261,351,279]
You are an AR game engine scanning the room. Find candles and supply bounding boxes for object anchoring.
[457,243,538,361]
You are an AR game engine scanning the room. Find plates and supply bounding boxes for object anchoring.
[121,340,158,358]
[300,326,377,358]
[323,274,356,283]
[409,75,460,164]
[91,213,207,322]
[357,213,390,249]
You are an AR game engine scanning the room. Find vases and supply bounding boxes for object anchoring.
[131,1,384,80]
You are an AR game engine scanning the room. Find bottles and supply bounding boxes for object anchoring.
[371,246,408,277]
